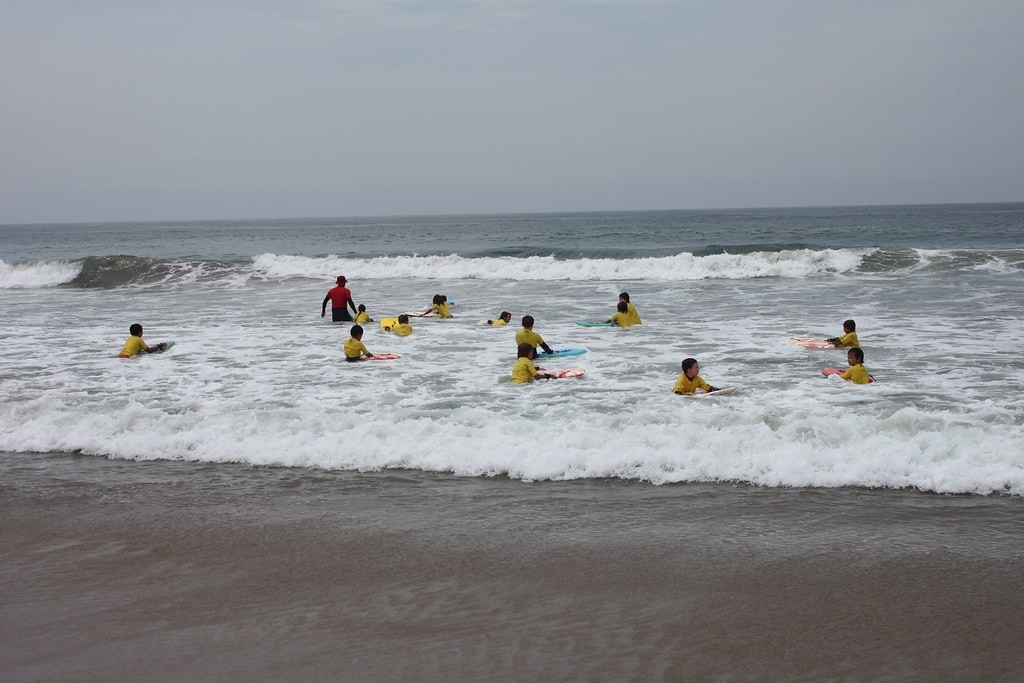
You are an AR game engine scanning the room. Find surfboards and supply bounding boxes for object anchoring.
[367,352,401,360]
[682,387,734,395]
[575,320,618,327]
[821,367,876,383]
[534,367,586,377]
[399,311,438,316]
[537,347,587,356]
[786,337,839,349]
[382,317,400,332]
[113,341,176,358]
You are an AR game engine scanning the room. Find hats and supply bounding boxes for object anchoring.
[336,275,348,284]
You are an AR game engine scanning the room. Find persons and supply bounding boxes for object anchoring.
[353,304,372,323]
[321,276,357,321]
[343,325,374,362]
[672,358,721,396]
[389,314,412,336]
[119,323,167,356]
[419,294,453,318]
[485,311,511,326]
[515,315,554,359]
[510,342,552,383]
[606,292,641,327]
[838,348,870,384]
[826,319,861,348]
